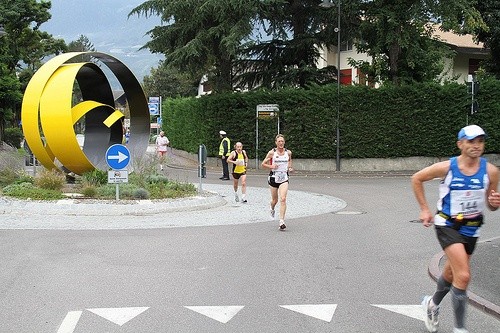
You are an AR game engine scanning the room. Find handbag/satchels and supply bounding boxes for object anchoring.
[455,212,483,227]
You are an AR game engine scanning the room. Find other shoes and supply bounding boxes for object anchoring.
[219,177,230,180]
[160,165,164,170]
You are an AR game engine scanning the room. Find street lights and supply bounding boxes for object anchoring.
[319,0,341,172]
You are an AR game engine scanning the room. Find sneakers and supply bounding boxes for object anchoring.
[278,222,286,230]
[270,206,275,217]
[421,295,440,333]
[241,195,247,202]
[235,193,239,202]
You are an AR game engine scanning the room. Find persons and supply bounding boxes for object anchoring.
[412,124,500,333]
[262,134,293,230]
[226,142,248,202]
[156,131,170,171]
[219,130,230,181]
[126,127,130,133]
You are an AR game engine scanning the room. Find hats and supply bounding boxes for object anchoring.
[457,125,487,141]
[219,131,226,134]
